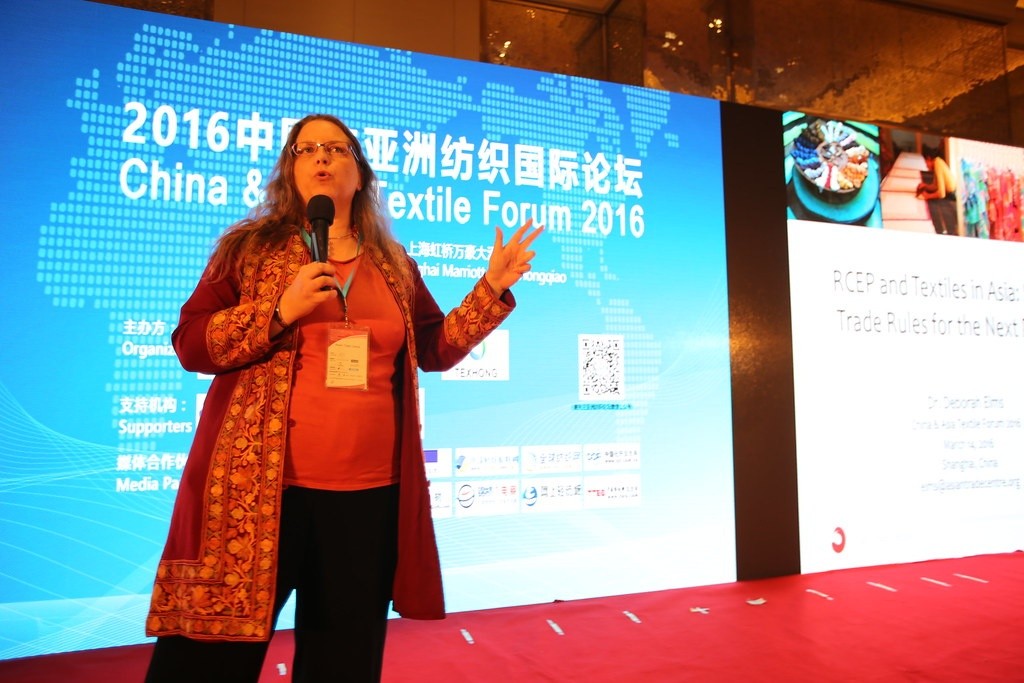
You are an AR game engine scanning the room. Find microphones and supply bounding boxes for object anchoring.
[306,195,335,291]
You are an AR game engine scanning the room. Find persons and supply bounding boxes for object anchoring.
[144,114,545,683]
[916,142,958,236]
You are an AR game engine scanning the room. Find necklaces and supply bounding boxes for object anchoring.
[328,232,353,239]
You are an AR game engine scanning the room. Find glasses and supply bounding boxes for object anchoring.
[290,141,351,157]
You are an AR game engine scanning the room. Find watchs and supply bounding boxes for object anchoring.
[272,303,288,329]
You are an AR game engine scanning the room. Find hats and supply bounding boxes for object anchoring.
[921,142,940,157]
